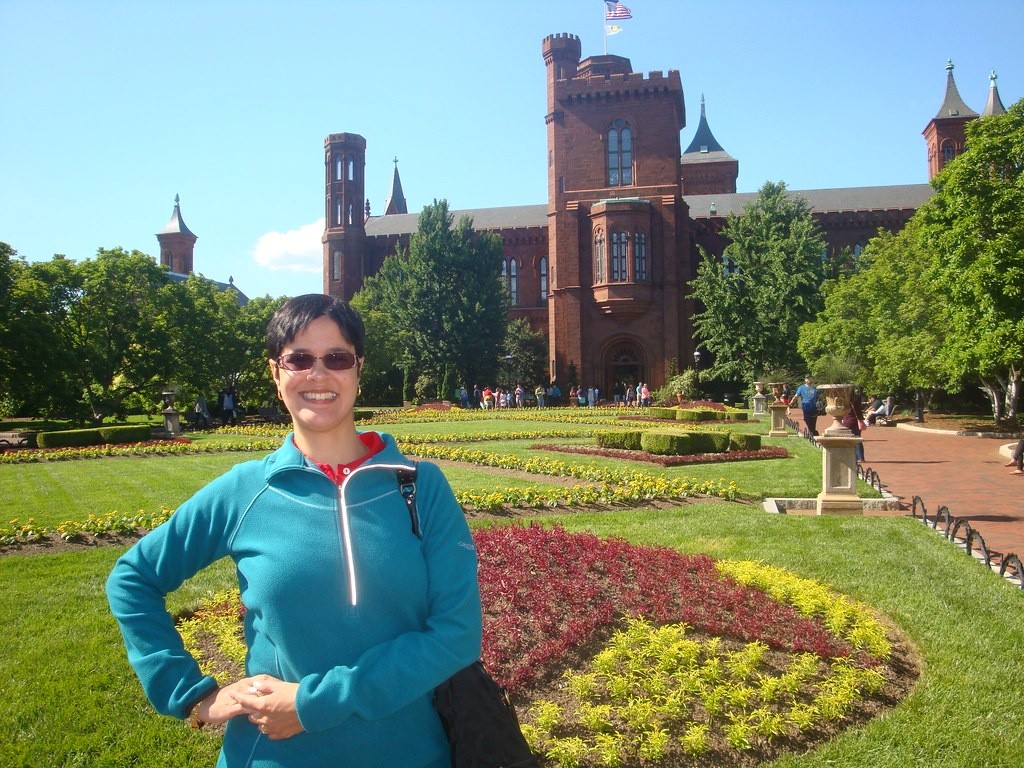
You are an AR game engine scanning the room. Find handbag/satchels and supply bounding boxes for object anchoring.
[397,460,538,768]
[858,420,867,431]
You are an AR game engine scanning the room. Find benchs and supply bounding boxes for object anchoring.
[876,405,898,427]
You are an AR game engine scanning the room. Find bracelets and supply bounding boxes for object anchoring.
[190,701,205,730]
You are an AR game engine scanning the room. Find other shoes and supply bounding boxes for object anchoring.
[1009,470,1024,476]
[1005,461,1017,466]
[856,459,864,463]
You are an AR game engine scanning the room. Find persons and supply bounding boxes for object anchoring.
[570,384,600,407]
[105,294,481,768]
[782,383,791,415]
[841,388,865,463]
[865,395,893,426]
[515,384,524,408]
[473,384,513,411]
[535,383,562,407]
[194,393,207,431]
[220,388,237,428]
[1005,439,1024,474]
[789,374,819,438]
[614,380,651,408]
[459,386,470,408]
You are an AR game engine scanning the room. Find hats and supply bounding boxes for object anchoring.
[805,374,812,379]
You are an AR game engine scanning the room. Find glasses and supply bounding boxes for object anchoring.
[275,353,359,371]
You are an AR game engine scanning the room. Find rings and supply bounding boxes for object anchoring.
[260,725,270,734]
[249,687,257,694]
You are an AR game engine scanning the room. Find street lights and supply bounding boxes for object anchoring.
[506,354,513,394]
[692,349,701,400]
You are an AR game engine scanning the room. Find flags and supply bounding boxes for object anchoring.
[606,2,632,20]
